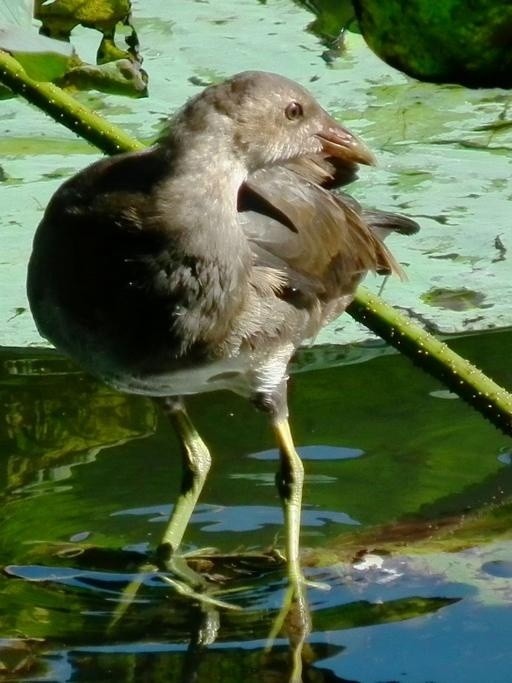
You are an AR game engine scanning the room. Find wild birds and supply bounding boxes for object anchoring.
[26,67,421,683]
